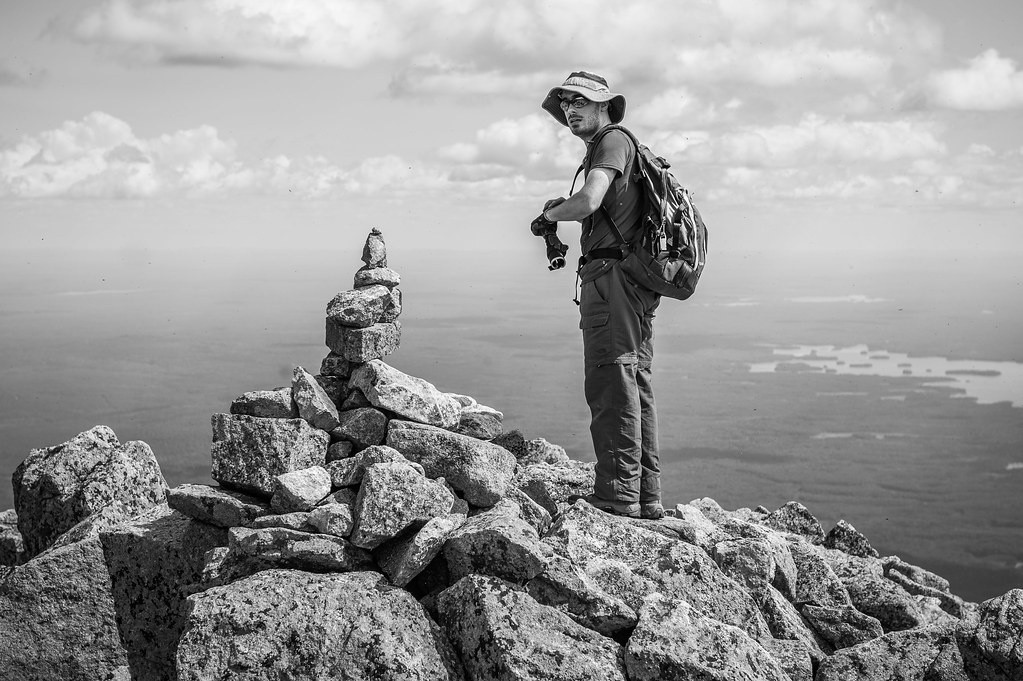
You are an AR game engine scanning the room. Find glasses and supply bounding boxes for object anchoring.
[560,95,592,110]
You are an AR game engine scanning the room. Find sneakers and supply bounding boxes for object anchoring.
[568,494,665,519]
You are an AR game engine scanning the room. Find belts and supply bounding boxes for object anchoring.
[578,247,625,264]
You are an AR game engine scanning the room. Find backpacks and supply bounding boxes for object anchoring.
[586,124,709,300]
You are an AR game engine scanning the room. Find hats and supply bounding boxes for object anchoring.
[541,70,627,128]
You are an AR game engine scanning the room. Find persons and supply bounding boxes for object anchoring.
[530,66,707,518]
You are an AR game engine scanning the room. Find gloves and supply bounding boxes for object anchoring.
[543,233,568,270]
[530,208,557,236]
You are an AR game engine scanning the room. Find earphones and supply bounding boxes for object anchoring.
[573,299,580,305]
